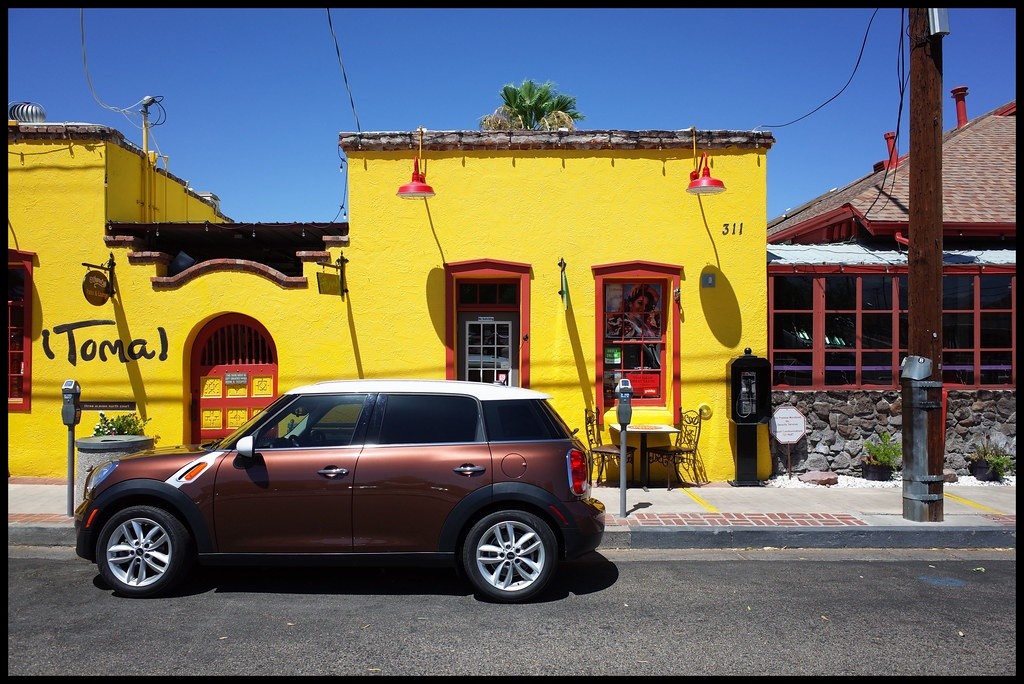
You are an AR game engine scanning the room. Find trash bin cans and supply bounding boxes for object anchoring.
[75,435,154,512]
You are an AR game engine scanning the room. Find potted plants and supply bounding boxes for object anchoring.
[860,430,904,483]
[964,435,1016,484]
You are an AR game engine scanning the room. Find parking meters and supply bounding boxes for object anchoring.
[615,378,634,517]
[62,379,82,517]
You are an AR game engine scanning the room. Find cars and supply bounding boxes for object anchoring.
[74,379,604,603]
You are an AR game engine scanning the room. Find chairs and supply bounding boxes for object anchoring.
[646,405,703,490]
[584,406,636,490]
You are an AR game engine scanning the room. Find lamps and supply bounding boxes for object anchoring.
[686,151,727,196]
[395,156,435,200]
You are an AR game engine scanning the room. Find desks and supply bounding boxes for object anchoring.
[607,424,682,488]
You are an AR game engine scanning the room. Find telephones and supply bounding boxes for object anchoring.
[741,371,756,401]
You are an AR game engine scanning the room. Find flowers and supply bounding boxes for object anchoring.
[89,409,153,435]
